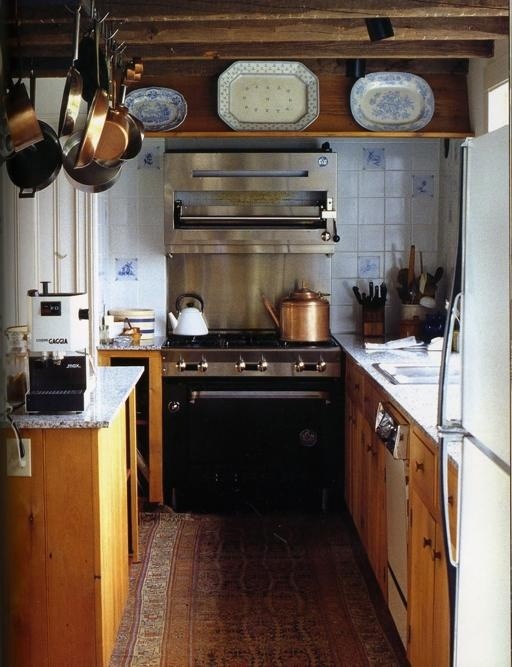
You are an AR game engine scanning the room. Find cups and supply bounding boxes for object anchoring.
[400,303,433,321]
[450,330,458,352]
[99,325,110,348]
[401,318,423,339]
[124,327,141,345]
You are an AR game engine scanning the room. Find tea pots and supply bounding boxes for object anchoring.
[167,292,210,336]
[261,278,332,342]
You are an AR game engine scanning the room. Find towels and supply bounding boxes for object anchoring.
[365,336,418,348]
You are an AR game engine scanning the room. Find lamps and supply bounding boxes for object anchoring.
[364,18,394,42]
[345,59,365,78]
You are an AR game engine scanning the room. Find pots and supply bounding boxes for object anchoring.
[1,0,144,198]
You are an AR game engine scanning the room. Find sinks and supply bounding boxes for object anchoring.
[373,359,462,387]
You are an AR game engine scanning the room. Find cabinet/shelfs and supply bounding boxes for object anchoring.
[0,387,138,667]
[345,358,458,667]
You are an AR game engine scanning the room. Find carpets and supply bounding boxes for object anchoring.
[105,511,411,667]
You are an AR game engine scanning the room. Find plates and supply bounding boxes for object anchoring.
[217,59,321,131]
[349,71,435,130]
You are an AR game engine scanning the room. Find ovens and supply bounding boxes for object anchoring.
[162,148,339,259]
[160,377,346,511]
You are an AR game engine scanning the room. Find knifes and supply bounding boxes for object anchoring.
[352,281,388,305]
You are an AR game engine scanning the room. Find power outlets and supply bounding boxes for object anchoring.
[6,439,32,477]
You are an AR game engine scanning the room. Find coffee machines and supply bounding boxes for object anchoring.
[25,281,91,415]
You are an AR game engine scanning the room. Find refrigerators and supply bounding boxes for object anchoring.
[433,123,512,666]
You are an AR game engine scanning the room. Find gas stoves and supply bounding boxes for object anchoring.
[160,330,344,378]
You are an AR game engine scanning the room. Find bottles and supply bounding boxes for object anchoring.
[423,313,443,342]
[4,324,30,406]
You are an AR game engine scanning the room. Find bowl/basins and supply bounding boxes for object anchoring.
[109,307,155,341]
[113,336,133,347]
[123,85,188,131]
